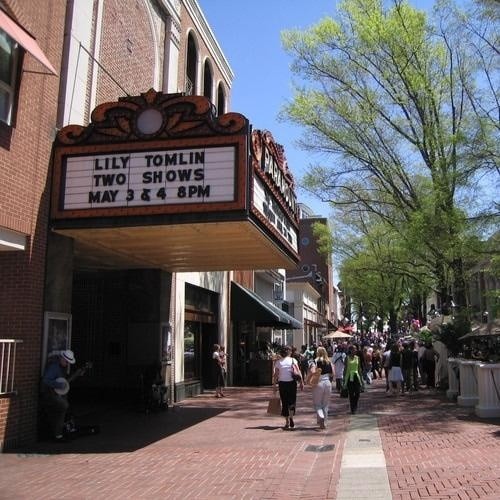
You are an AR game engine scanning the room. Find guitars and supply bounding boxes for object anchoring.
[48,362,93,399]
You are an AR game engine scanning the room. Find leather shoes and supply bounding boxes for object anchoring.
[285,416,326,429]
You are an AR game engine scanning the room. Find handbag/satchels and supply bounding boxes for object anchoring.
[341,382,348,398]
[267,391,282,415]
[305,361,322,388]
[291,358,302,382]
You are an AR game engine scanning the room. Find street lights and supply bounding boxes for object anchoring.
[341,300,382,375]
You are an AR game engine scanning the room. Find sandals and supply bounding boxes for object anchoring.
[220,392,225,397]
[215,393,222,398]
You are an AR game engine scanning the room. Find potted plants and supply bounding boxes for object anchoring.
[432,318,500,419]
[256,336,283,385]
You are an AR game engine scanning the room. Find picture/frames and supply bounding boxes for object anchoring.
[157,320,174,367]
[41,310,73,380]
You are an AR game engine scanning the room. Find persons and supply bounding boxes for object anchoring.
[39,350,76,443]
[273,297,500,429]
[212,343,225,398]
[219,346,225,368]
